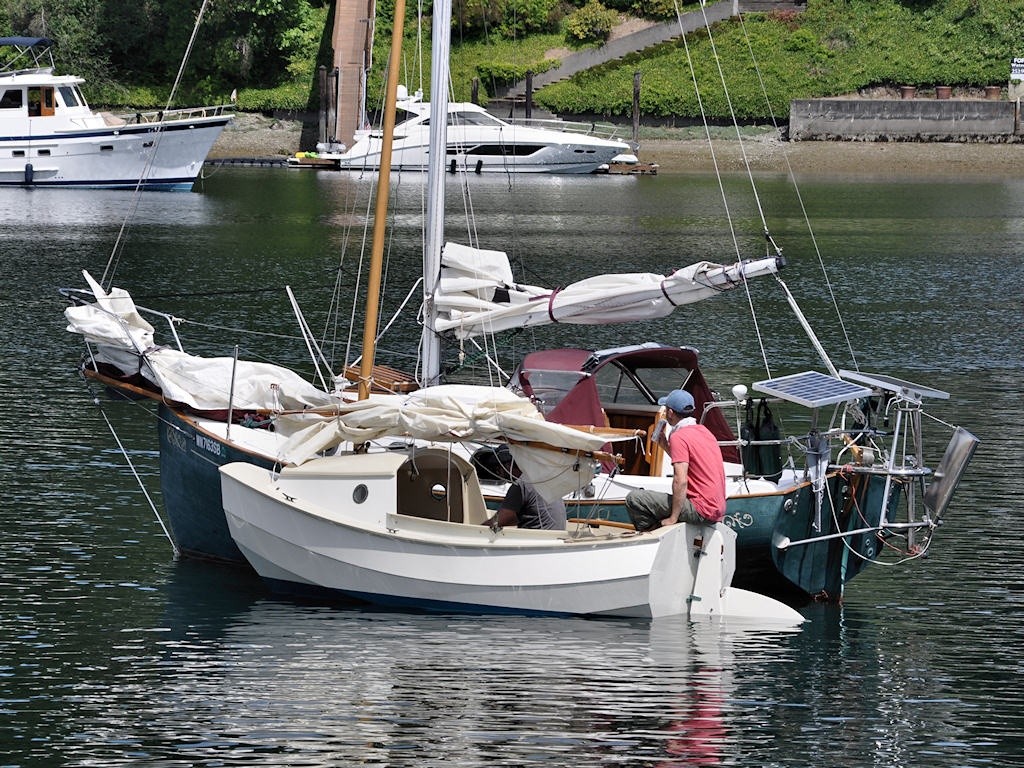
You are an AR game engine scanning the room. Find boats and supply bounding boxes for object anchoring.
[288,0,639,175]
[0,37,239,190]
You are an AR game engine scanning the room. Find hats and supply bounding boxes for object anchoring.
[657,390,695,414]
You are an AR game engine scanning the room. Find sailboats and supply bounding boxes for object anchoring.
[215,1,741,619]
[57,0,980,601]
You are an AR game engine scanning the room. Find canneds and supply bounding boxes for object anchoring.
[651,418,666,443]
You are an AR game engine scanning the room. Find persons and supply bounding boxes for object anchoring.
[480,470,567,531]
[625,388,725,533]
